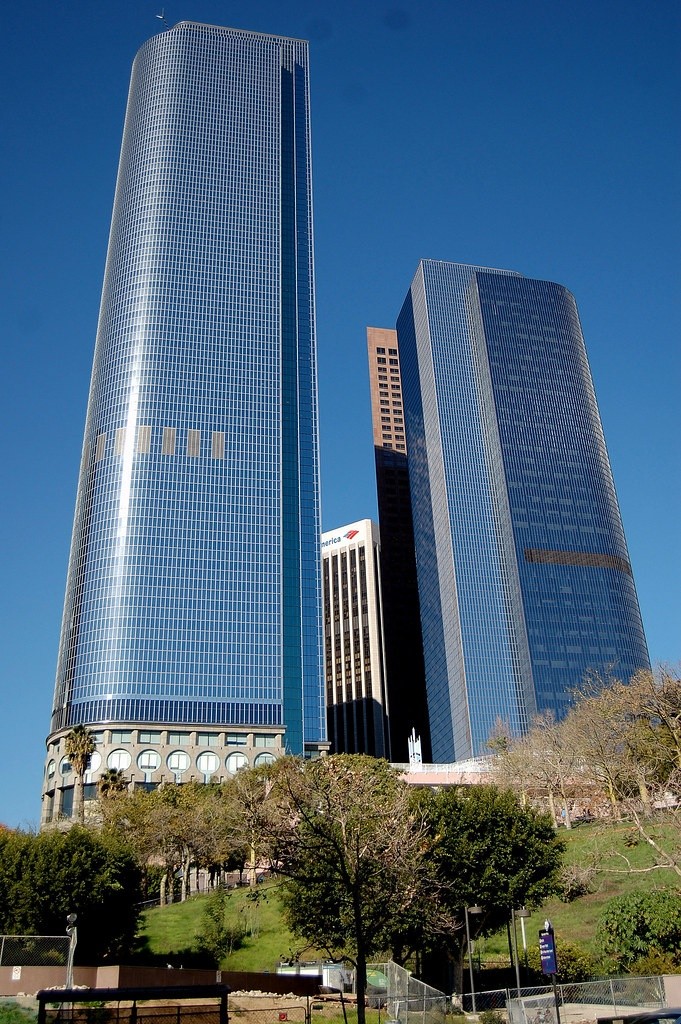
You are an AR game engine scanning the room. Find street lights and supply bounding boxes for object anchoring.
[464,903,484,1013]
[511,907,532,1001]
